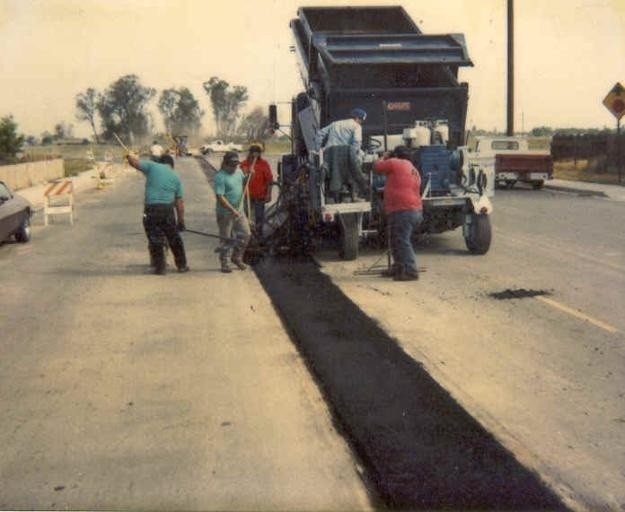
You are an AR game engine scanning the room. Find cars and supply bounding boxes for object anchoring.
[1,180,34,244]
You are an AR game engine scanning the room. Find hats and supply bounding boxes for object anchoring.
[351,108,367,120]
[223,152,240,165]
[249,145,261,151]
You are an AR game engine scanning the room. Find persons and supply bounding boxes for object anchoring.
[373,145,425,282]
[240,145,274,239]
[314,107,368,202]
[149,140,162,157]
[213,152,252,273]
[126,150,191,274]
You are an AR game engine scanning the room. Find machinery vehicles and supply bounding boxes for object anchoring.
[278,3,494,263]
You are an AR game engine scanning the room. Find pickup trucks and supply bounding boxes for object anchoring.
[201,139,244,154]
[469,131,556,185]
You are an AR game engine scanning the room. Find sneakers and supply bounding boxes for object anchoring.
[396,273,417,280]
[156,259,247,275]
[383,266,399,275]
